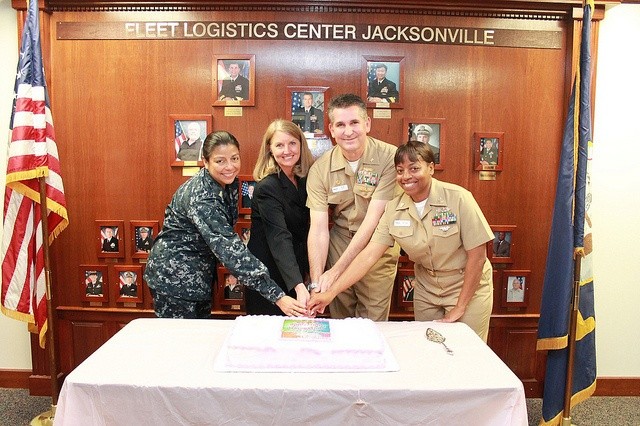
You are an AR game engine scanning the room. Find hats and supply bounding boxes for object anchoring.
[124,271,134,278]
[139,227,149,233]
[414,124,433,136]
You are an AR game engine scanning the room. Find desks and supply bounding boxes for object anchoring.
[46,317,529,426]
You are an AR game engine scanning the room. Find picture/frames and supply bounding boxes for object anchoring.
[359,54,406,109]
[285,85,330,138]
[236,175,260,216]
[94,219,126,259]
[236,219,252,243]
[111,265,144,304]
[77,264,110,302]
[214,266,245,304]
[499,269,532,309]
[395,268,417,307]
[488,225,518,263]
[472,130,505,173]
[401,116,447,171]
[128,219,159,258]
[167,112,213,167]
[210,54,255,107]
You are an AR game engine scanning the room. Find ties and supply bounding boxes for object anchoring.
[305,110,309,131]
[497,240,501,251]
[108,240,109,242]
[378,82,380,85]
[232,80,233,83]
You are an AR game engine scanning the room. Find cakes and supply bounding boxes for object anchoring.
[221,314,386,370]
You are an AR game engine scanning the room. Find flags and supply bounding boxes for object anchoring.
[292,93,301,114]
[516,277,524,288]
[408,123,415,140]
[242,181,249,197]
[174,120,187,162]
[480,139,484,154]
[369,65,377,80]
[403,276,413,300]
[535,0,597,425]
[120,274,127,291]
[1,0,69,349]
[101,230,105,250]
[85,273,90,287]
[136,228,140,246]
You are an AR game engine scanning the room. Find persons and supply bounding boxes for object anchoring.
[244,120,314,314]
[218,59,250,100]
[85,271,104,297]
[491,231,510,256]
[413,124,440,163]
[175,120,207,161]
[309,142,494,346]
[100,226,119,252]
[306,96,406,323]
[508,277,524,302]
[136,227,153,252]
[368,64,399,104]
[142,130,308,319]
[120,271,137,298]
[292,92,323,132]
[481,140,497,164]
[405,280,415,302]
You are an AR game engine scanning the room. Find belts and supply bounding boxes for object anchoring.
[421,267,464,278]
[332,224,356,238]
